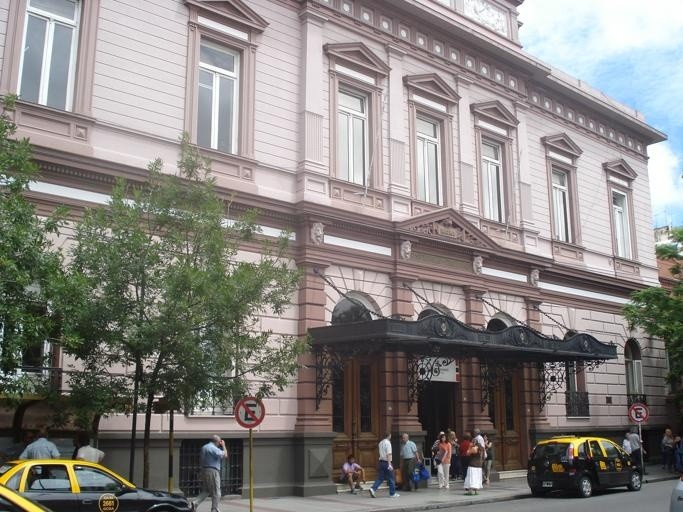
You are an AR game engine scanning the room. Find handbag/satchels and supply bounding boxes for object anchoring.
[412,462,430,481]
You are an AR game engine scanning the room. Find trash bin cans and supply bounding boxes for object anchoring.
[423,458,432,477]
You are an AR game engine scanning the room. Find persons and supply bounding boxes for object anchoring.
[369,433,400,498]
[628,426,648,474]
[431,427,493,495]
[191,435,227,512]
[339,455,367,495]
[623,432,631,456]
[662,429,683,481]
[400,433,422,491]
[3,424,105,463]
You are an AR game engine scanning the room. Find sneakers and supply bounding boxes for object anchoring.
[391,494,399,497]
[369,489,376,498]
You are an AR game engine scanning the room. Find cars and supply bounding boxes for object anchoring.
[0,484,53,511]
[0,459,196,512]
[527,434,642,496]
